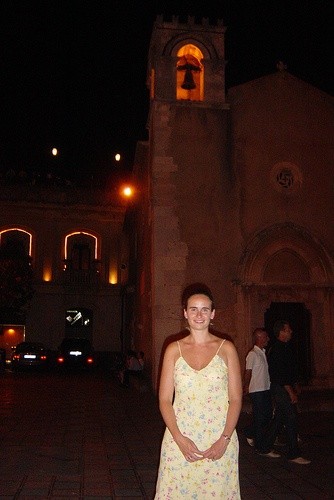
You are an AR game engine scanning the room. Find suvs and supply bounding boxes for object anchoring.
[54,337,97,375]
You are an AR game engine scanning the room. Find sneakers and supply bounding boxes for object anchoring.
[245,436,255,447]
[257,450,280,457]
[289,457,311,464]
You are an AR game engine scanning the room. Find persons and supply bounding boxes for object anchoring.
[154,289,242,500]
[244,320,312,465]
[116,350,147,388]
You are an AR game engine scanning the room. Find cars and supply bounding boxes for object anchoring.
[9,342,52,372]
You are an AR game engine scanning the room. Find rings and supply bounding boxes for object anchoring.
[213,456,217,459]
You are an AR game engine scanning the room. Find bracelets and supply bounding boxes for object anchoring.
[221,433,231,440]
